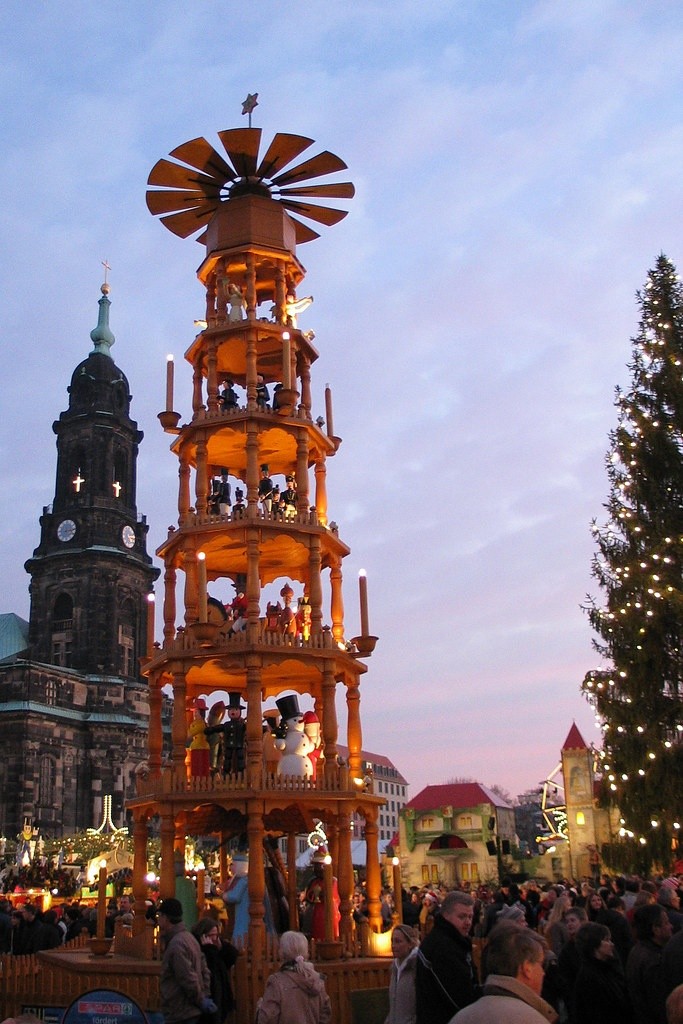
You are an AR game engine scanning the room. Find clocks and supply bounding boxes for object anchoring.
[58,519,76,542]
[122,526,136,549]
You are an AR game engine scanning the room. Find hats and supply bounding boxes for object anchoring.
[155,898,183,916]
[65,908,78,921]
[662,877,679,890]
[425,889,442,903]
[552,886,564,897]
[496,903,525,922]
[500,876,512,887]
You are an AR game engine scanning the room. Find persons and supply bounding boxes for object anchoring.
[180,283,342,951]
[0,874,683,1024]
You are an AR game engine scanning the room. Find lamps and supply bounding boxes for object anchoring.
[89,859,115,955]
[316,855,345,960]
[392,856,403,926]
